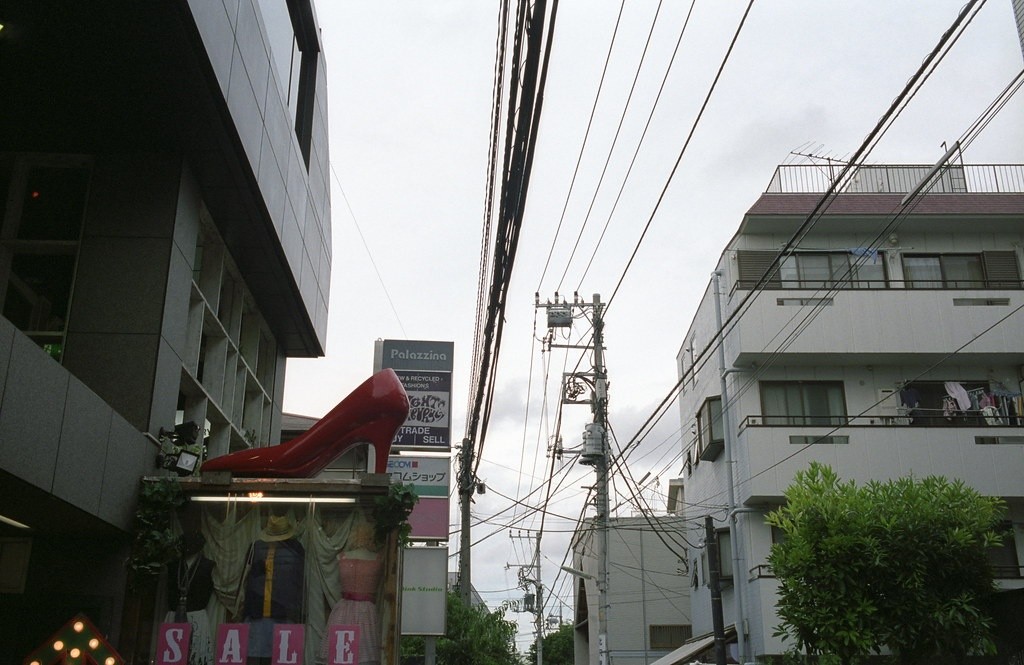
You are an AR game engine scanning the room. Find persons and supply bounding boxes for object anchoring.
[316,545,383,665]
[239,537,305,665]
[165,552,215,665]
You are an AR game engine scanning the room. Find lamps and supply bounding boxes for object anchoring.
[159,421,200,447]
[155,449,200,477]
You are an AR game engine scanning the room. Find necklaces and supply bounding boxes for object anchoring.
[173,554,202,623]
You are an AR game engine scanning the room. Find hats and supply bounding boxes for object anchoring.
[259,512,296,543]
[177,528,206,554]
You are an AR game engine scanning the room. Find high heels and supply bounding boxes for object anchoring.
[198,367,411,478]
